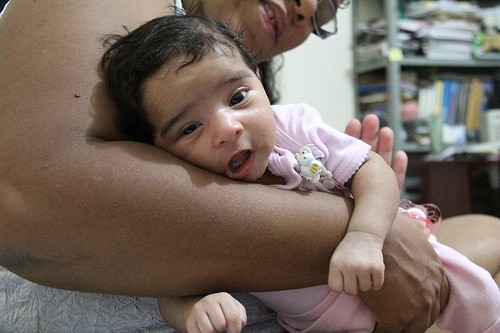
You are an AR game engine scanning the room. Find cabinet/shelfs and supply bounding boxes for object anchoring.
[348,0,500,197]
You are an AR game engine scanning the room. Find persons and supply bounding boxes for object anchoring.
[98,2,500,333]
[1,1,500,333]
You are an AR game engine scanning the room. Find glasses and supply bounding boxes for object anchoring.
[312,0,338,39]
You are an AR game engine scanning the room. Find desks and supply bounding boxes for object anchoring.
[418,155,499,227]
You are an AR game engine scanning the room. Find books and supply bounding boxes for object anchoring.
[354,2,500,160]
